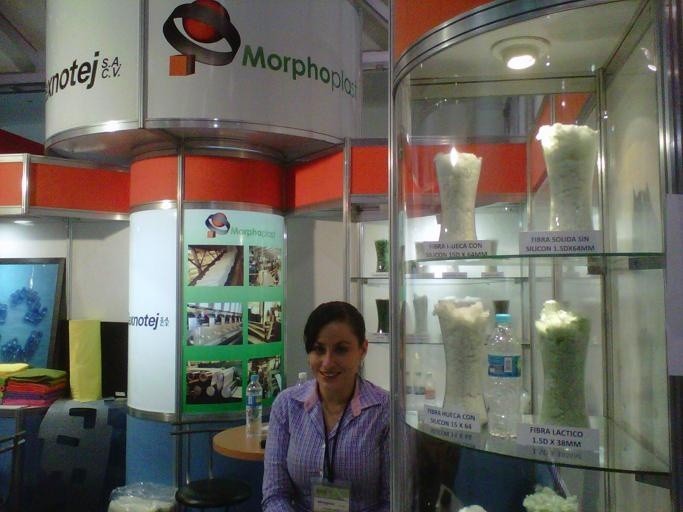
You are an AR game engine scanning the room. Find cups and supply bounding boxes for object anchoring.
[530,300,593,427]
[436,130,484,240]
[537,122,601,233]
[374,240,510,336]
[435,296,488,414]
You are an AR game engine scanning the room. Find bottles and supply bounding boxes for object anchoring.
[245,375,262,441]
[484,315,524,436]
[296,372,307,387]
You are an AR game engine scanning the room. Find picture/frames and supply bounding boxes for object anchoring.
[0,257,66,369]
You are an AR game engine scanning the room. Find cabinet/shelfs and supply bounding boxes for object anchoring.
[388,2,676,511]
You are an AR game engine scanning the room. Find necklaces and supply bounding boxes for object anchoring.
[320,397,347,415]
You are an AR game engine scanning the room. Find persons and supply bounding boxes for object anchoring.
[257,300,422,511]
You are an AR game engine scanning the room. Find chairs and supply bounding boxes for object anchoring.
[170,418,252,511]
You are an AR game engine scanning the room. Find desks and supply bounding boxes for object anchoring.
[0,403,50,512]
